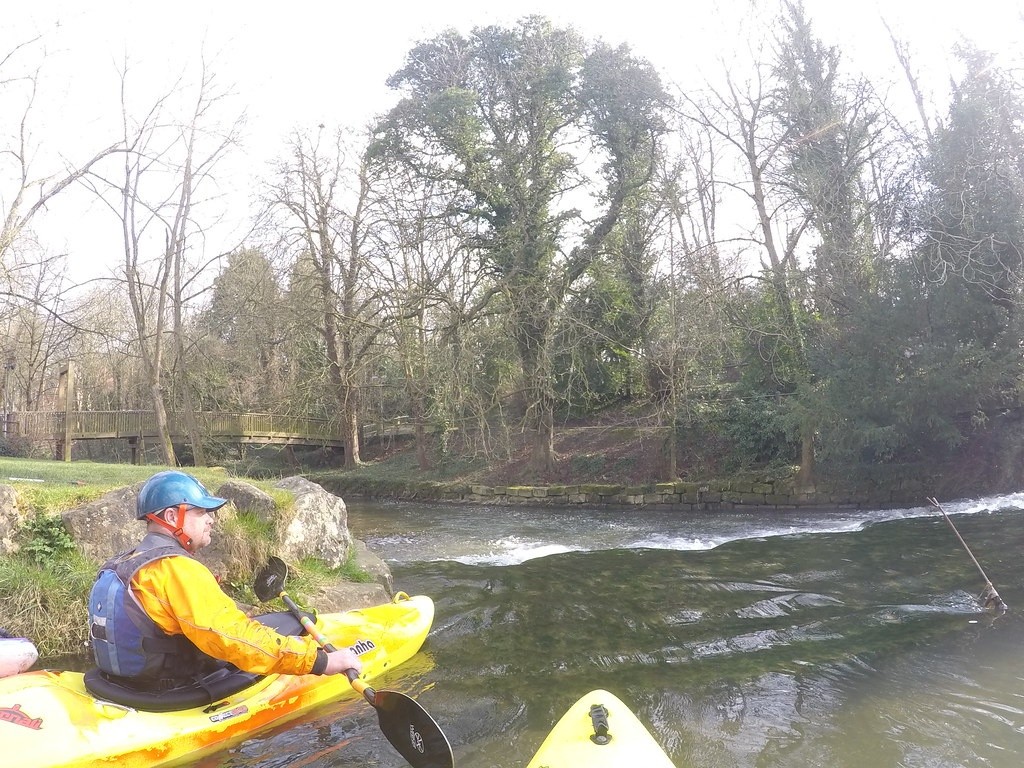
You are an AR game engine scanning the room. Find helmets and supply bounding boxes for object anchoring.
[136,469,226,520]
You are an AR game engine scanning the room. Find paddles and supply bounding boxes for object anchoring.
[253,555,455,768]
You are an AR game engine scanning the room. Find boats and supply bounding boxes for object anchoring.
[0,588,434,767]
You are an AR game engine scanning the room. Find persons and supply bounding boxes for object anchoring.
[89,470,363,686]
